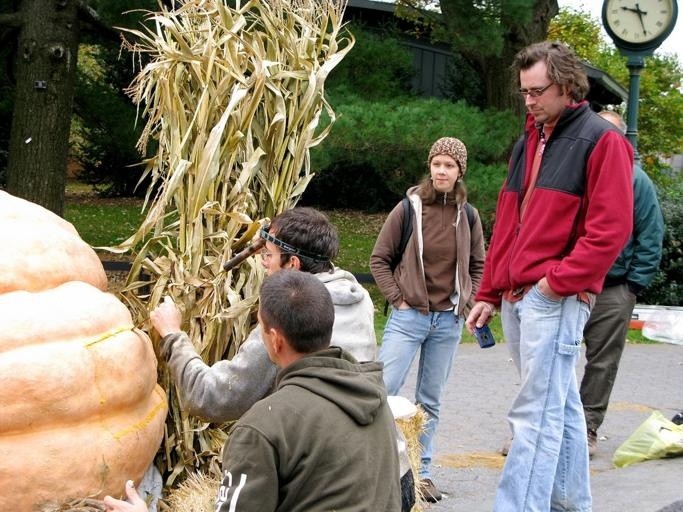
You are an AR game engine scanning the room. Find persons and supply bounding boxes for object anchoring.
[463,38,637,512]
[147,200,417,512]
[495,107,665,460]
[363,135,488,504]
[101,266,404,512]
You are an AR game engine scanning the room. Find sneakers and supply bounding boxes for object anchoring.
[419,478,441,501]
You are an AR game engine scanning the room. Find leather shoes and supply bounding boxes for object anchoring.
[587,429,597,457]
[502,438,513,456]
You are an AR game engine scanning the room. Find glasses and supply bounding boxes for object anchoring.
[516,81,555,98]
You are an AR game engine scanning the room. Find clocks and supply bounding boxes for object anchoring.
[602,2,680,51]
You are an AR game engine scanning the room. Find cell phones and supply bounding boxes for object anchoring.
[474,324,495,348]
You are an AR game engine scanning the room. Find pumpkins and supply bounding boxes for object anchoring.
[0,187,170,512]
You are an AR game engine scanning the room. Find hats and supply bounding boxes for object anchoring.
[426,137,468,178]
